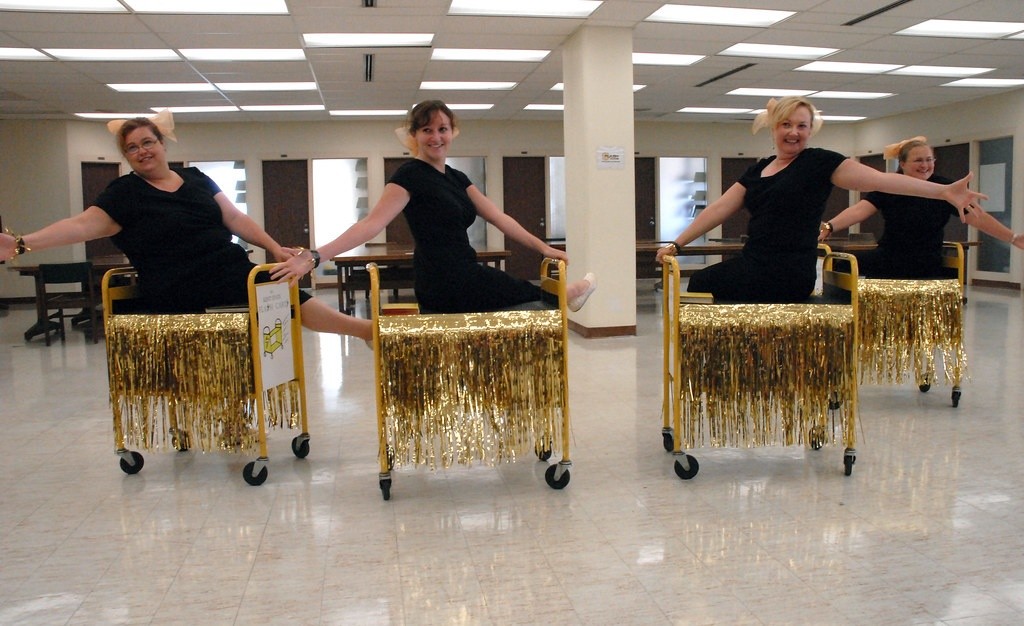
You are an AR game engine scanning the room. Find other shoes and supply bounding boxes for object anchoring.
[568,272,596,311]
[365,339,374,351]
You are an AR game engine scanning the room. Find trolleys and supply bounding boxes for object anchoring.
[815,242,964,407]
[659,251,858,479]
[365,258,570,501]
[102,265,310,487]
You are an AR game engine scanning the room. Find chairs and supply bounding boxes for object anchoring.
[366,243,400,298]
[37,262,103,347]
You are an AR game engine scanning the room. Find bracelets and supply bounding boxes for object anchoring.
[665,242,681,255]
[1010,232,1018,244]
[823,221,833,234]
[310,250,320,269]
[14,237,27,254]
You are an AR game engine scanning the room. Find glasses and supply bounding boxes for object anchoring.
[906,157,936,165]
[124,137,158,155]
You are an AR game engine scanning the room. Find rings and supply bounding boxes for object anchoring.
[966,205,971,211]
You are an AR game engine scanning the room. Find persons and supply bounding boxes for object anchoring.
[268,99,596,316]
[816,135,1024,280]
[1,116,375,340]
[655,96,991,304]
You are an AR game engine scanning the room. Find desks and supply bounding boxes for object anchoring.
[8,254,140,343]
[543,234,985,306]
[330,244,513,316]
